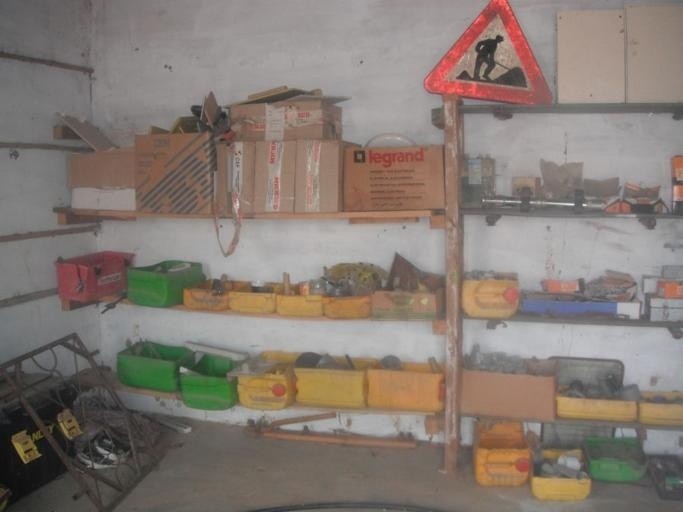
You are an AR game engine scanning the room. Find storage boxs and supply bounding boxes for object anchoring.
[227,281,279,317]
[124,258,204,308]
[294,138,362,213]
[371,281,443,318]
[461,368,682,501]
[114,340,193,393]
[235,349,300,411]
[292,354,371,411]
[66,147,134,212]
[461,278,518,319]
[341,143,446,213]
[133,124,212,213]
[276,292,322,318]
[366,358,444,413]
[181,278,247,313]
[177,352,237,411]
[59,252,136,305]
[213,139,255,214]
[227,101,344,141]
[321,293,370,318]
[253,139,297,215]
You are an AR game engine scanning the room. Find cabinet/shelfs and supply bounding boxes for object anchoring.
[51,92,683,473]
